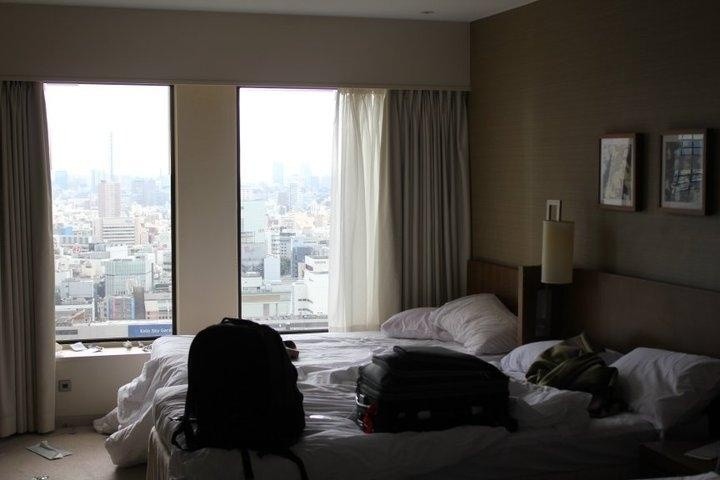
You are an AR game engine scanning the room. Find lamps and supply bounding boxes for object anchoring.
[539,220,575,294]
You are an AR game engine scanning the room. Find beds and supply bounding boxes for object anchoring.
[146,262,718,480]
[92,254,524,468]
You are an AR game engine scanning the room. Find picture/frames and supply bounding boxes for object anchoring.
[597,132,642,214]
[657,125,711,218]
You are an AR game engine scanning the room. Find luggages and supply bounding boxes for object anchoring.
[354,346,517,434]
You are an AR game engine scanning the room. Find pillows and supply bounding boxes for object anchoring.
[490,338,621,378]
[598,343,719,437]
[428,288,523,360]
[380,303,455,342]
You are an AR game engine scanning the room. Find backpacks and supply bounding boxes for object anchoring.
[171,318,307,480]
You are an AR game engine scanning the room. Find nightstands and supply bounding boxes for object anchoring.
[634,438,719,479]
